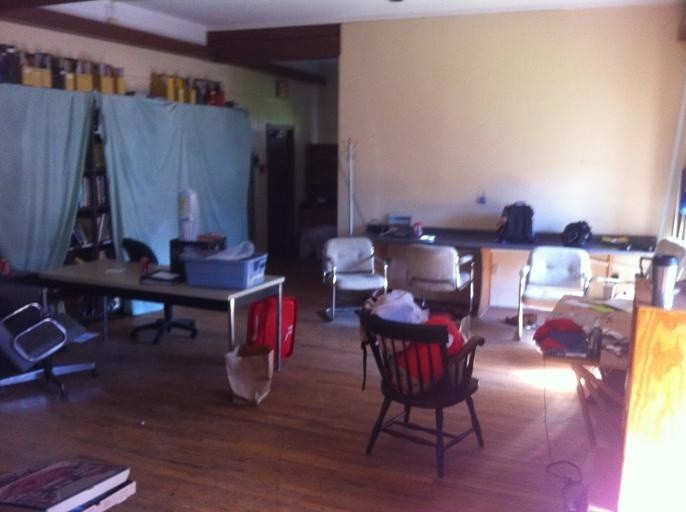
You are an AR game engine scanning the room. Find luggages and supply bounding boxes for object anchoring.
[246,294,297,361]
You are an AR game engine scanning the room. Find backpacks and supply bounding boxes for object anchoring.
[496,200,534,244]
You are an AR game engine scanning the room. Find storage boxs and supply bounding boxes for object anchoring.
[169,237,269,291]
[246,296,298,368]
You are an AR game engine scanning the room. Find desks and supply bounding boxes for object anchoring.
[359,224,657,321]
[18,259,286,398]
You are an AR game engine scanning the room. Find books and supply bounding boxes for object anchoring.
[47,140,131,323]
[68,480,137,512]
[139,269,185,288]
[0,452,132,512]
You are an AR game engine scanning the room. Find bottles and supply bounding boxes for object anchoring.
[413,220,423,236]
[140,257,150,275]
[652,254,678,311]
[13,41,122,76]
[178,187,199,243]
[155,65,227,108]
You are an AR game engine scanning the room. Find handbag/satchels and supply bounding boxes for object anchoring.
[362,285,427,358]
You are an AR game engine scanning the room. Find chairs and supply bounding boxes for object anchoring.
[323,236,476,322]
[119,236,199,344]
[516,238,686,364]
[352,310,489,478]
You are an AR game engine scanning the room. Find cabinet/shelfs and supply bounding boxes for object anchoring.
[52,97,128,325]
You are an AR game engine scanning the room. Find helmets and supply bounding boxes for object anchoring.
[561,221,591,247]
